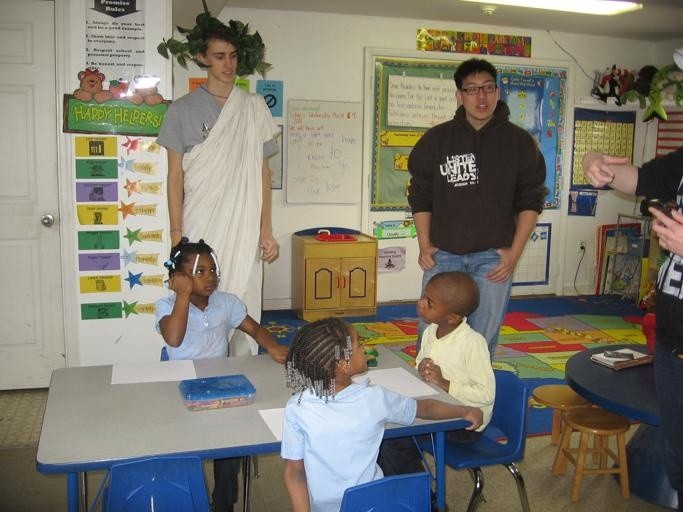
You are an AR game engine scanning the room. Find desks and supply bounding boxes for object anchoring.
[566,338,681,510]
[35,344,474,512]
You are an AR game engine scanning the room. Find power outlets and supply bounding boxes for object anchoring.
[575,240,586,254]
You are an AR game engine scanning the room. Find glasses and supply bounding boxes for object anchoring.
[460,85,497,96]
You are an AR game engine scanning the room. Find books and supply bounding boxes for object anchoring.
[587,346,654,372]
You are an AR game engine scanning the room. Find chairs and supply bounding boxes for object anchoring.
[160,348,250,512]
[338,472,432,512]
[419,371,530,511]
[100,455,213,512]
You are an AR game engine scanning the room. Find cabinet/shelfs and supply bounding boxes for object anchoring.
[291,240,379,322]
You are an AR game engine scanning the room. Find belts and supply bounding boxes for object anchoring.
[435,246,488,254]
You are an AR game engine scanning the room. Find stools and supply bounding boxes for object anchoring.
[527,385,594,446]
[550,407,630,501]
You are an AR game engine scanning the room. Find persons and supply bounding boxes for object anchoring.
[582,147,683,511]
[407,60,546,364]
[378,270,496,511]
[280,316,484,511]
[154,237,291,511]
[154,28,280,356]
